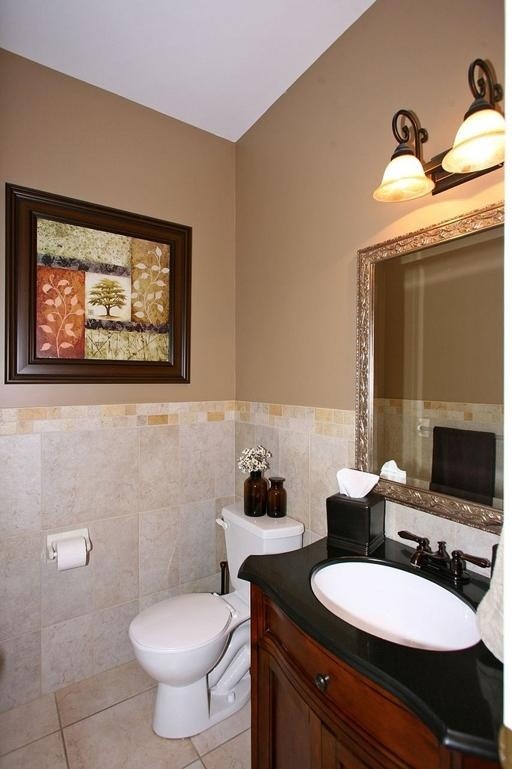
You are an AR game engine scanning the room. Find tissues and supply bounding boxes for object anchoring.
[326,469,385,556]
[380,460,407,484]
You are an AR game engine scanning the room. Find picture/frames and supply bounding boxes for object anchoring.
[5,180,193,385]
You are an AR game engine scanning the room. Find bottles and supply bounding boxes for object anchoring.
[265,477,287,519]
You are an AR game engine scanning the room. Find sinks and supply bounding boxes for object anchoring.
[310,556,481,651]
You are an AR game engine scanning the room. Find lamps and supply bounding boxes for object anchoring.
[373,58,505,203]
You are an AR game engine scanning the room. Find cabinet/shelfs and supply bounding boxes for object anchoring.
[249,582,502,769]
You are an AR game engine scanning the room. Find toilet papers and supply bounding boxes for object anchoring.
[54,537,87,571]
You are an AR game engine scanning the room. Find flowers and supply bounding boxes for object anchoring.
[236,444,272,474]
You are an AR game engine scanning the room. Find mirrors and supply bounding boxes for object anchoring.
[355,201,504,535]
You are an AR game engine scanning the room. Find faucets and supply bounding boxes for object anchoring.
[410,541,450,570]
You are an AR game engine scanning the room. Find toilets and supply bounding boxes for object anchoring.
[128,501,304,738]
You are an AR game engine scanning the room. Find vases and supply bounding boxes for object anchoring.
[244,472,267,517]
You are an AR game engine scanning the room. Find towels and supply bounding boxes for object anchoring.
[475,522,503,667]
